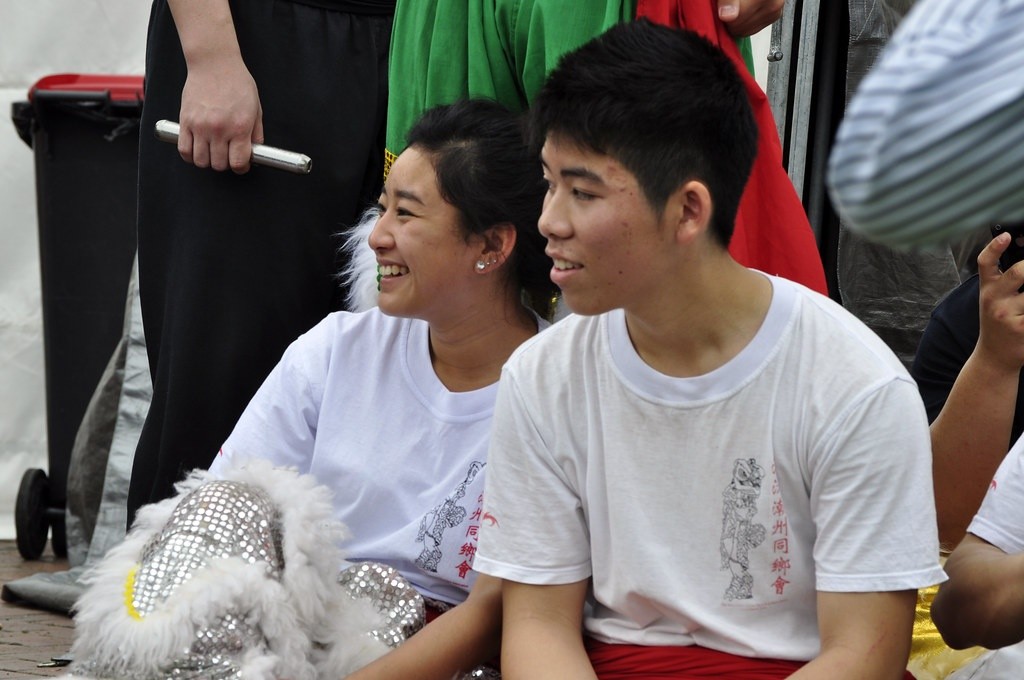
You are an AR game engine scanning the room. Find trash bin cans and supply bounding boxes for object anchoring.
[11,71,147,561]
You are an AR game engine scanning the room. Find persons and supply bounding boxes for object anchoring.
[909,231,1024,553]
[827,0,1024,255]
[929,431,1024,680]
[125,0,397,535]
[472,17,949,680]
[382,0,828,296]
[202,100,561,680]
